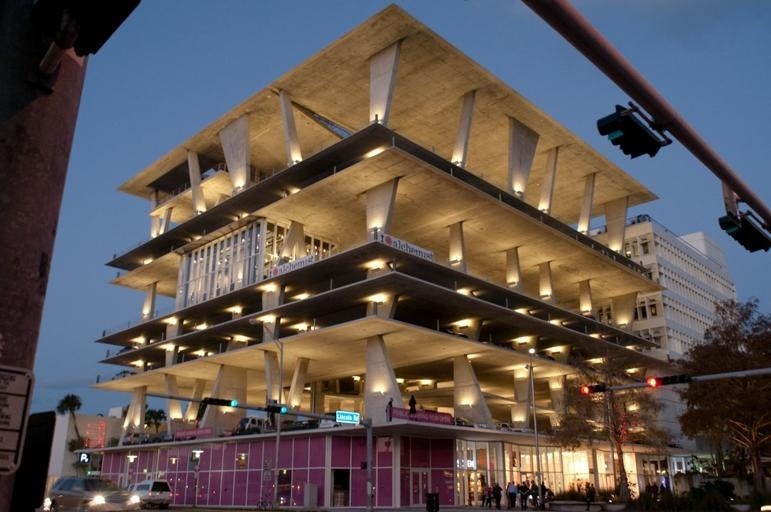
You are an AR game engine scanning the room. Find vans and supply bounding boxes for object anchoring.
[235,416,264,433]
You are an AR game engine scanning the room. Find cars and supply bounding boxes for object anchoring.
[43,475,173,512]
[529,488,554,505]
[280,411,339,430]
[123,431,174,444]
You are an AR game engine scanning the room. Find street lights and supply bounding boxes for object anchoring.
[127,455,137,485]
[249,318,283,507]
[528,348,545,510]
[192,450,204,507]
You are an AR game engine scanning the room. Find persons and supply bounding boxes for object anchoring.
[653,480,660,506]
[480,480,556,511]
[409,393,418,420]
[646,481,651,498]
[659,482,666,505]
[586,481,593,511]
[387,396,395,423]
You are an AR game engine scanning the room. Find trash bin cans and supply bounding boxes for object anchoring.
[334,487,345,507]
[426,492,439,512]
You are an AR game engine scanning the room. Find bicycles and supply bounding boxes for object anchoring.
[257,494,273,511]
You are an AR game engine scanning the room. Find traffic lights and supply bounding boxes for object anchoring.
[581,384,606,395]
[204,398,238,407]
[265,405,287,414]
[719,211,771,253]
[597,101,672,159]
[647,372,691,388]
